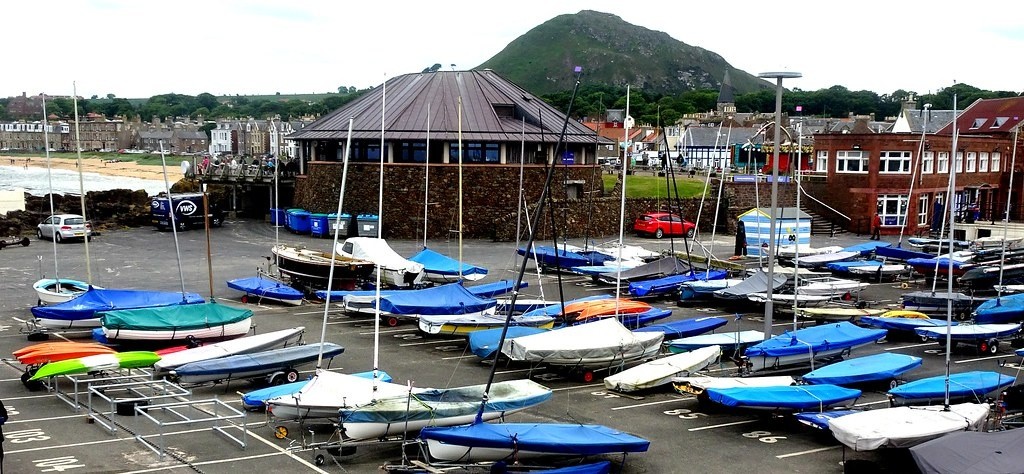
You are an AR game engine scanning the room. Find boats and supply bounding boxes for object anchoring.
[16,70,1024,474]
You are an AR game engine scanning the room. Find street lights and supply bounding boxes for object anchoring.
[759,73,801,339]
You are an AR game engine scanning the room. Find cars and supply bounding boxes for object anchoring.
[635,212,696,239]
[37,214,91,243]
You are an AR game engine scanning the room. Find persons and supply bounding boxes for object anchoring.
[74,158,120,167]
[642,152,648,166]
[10,157,30,169]
[197,154,279,175]
[677,154,684,167]
[659,154,666,170]
[869,213,880,240]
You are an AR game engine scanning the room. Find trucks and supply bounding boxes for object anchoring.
[150,193,223,230]
